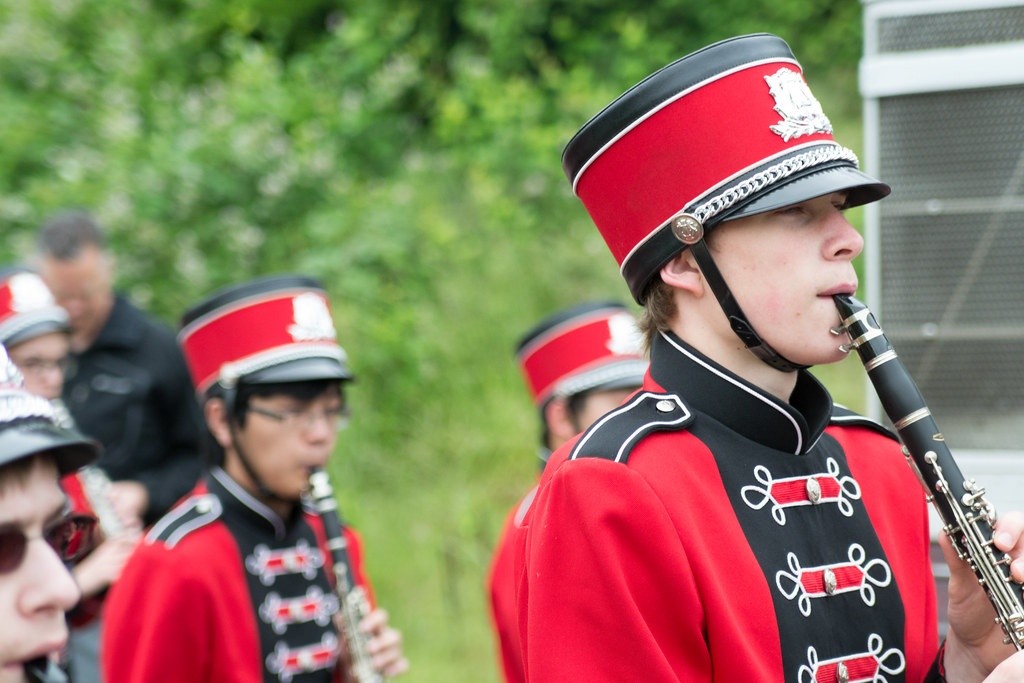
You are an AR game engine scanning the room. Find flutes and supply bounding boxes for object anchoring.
[831,292,1024,650]
[301,467,385,682]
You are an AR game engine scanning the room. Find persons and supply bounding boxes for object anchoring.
[488,301,650,683]
[0,345,100,683]
[101,278,409,683]
[527,34,1024,683]
[37,215,210,524]
[0,270,141,683]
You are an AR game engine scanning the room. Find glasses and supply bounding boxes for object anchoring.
[0,514,100,567]
[243,404,348,434]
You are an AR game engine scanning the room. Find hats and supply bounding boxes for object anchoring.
[175,276,353,410]
[561,31,891,307]
[512,300,651,414]
[0,345,102,480]
[0,267,78,348]
